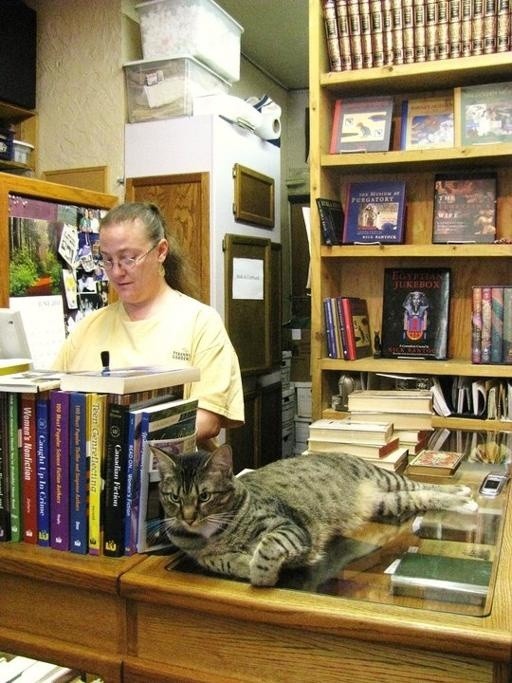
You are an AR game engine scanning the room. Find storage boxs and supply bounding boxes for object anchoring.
[1,126,35,163]
[282,328,310,458]
[122,55,232,122]
[137,1,245,82]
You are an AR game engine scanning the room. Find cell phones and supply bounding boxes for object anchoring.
[479,474,506,494]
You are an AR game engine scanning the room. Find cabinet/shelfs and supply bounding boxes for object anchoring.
[310,0,510,459]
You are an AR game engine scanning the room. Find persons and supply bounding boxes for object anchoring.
[50,201,246,447]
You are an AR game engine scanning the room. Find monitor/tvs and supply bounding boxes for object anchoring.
[0,309,32,361]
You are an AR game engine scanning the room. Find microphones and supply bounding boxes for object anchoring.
[98,351,110,366]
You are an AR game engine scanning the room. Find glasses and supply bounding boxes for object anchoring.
[95,240,161,271]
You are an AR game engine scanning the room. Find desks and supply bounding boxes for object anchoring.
[2,536,149,683]
[120,474,511,682]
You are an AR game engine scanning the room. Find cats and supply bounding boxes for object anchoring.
[145,442,476,589]
